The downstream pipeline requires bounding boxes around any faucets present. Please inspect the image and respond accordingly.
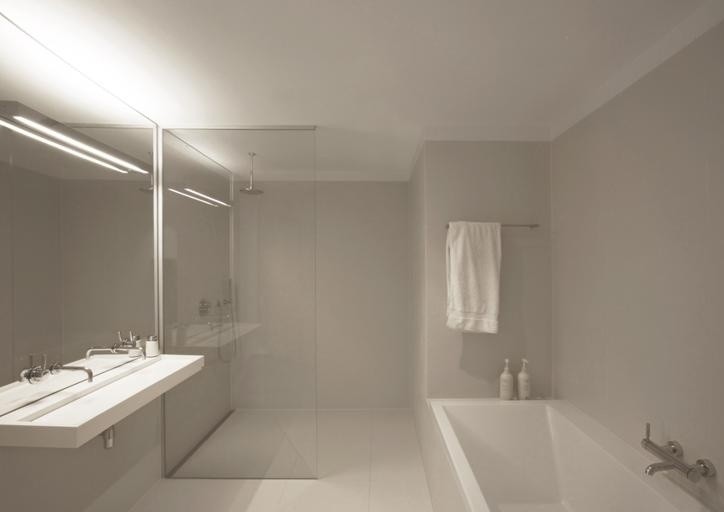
[637,421,716,484]
[208,322,223,331]
[115,343,146,359]
[20,362,94,383]
[85,345,118,359]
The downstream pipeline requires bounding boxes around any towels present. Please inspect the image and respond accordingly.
[443,217,502,334]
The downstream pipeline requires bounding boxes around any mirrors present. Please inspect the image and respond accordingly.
[161,127,232,354]
[0,9,154,394]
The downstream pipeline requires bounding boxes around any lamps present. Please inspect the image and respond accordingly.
[0,117,128,177]
[167,184,217,211]
[184,184,231,208]
[4,100,153,175]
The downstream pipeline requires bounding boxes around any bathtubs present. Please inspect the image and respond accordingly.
[427,398,723,512]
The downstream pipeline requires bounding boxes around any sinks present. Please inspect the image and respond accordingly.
[187,321,262,361]
[185,322,222,340]
[0,353,142,417]
[0,352,205,449]
